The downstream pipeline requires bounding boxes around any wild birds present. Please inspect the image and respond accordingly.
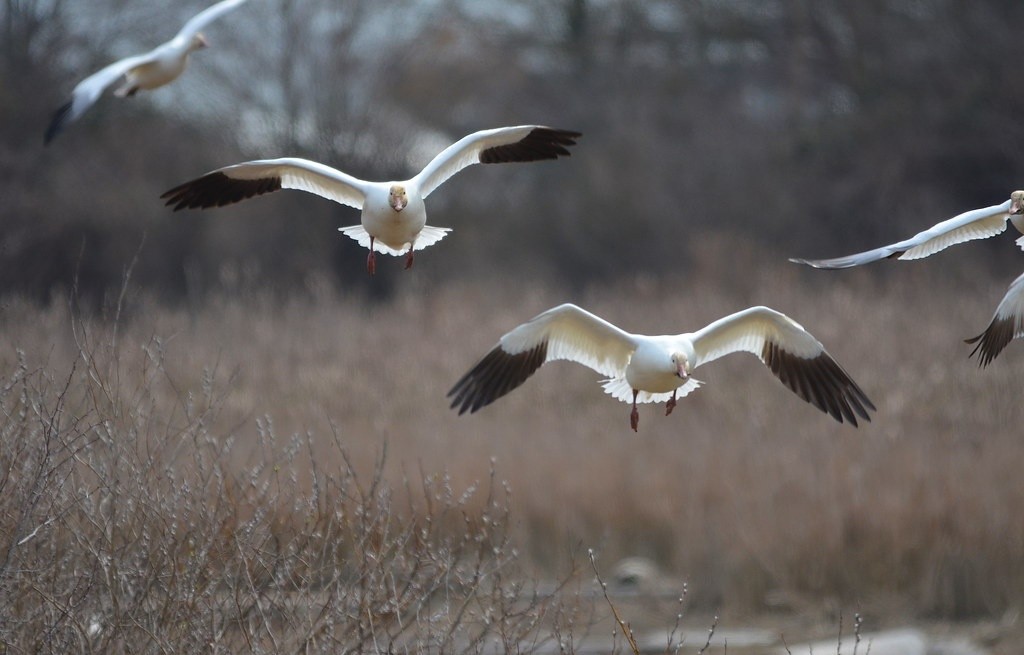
[41,0,249,148]
[160,125,583,278]
[446,303,877,430]
[789,190,1024,370]
